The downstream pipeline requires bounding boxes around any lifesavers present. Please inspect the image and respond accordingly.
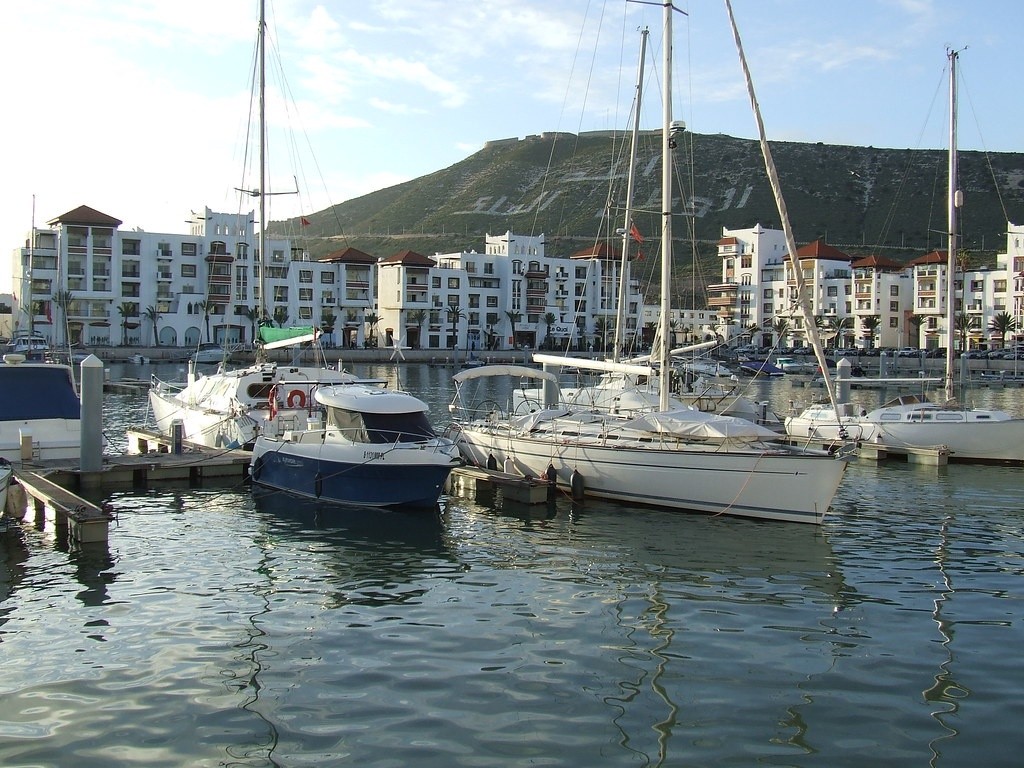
[287,390,306,408]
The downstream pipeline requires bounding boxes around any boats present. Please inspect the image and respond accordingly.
[5,329,50,354]
[126,352,151,364]
[245,378,464,514]
[188,342,233,365]
[0,456,14,513]
[0,195,109,461]
[68,352,89,364]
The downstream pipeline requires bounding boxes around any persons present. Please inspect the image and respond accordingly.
[171,336,191,345]
[90,335,110,346]
[128,336,139,344]
[472,340,475,351]
[219,337,239,343]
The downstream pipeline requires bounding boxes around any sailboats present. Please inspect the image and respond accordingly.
[426,1,861,526]
[146,0,409,453]
[783,44,1024,467]
[512,26,838,449]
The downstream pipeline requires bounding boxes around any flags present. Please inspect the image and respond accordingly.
[301,216,310,226]
[631,223,642,243]
[638,250,643,258]
